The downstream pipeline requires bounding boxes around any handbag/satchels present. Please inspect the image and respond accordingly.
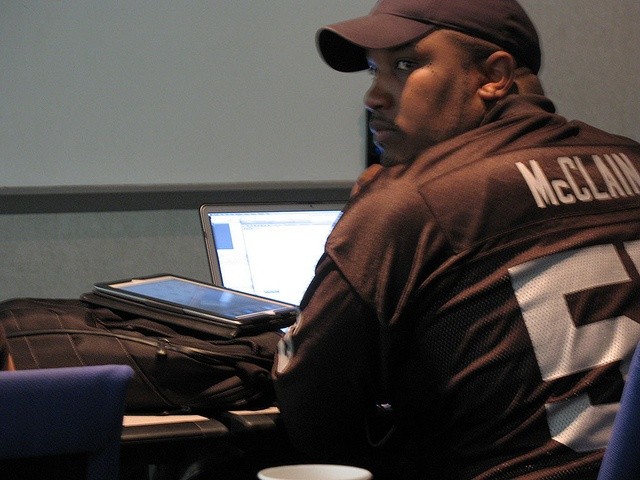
[1,298,286,417]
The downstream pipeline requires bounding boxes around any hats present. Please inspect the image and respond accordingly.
[314,0,541,75]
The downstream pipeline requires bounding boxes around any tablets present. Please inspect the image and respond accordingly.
[200,200,349,306]
[93,269,299,337]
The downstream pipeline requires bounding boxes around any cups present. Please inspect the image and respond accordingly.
[258,464,372,478]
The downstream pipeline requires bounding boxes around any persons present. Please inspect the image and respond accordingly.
[277,1,640,479]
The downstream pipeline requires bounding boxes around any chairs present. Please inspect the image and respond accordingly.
[598,343,639,478]
[0,365,134,480]
